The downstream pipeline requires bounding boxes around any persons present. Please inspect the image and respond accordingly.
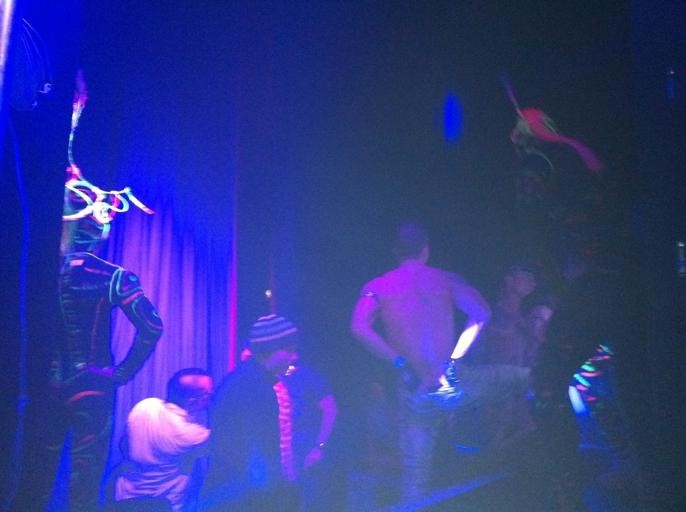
[3,107,686,510]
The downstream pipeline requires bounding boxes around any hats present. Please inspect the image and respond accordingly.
[247,313,299,354]
